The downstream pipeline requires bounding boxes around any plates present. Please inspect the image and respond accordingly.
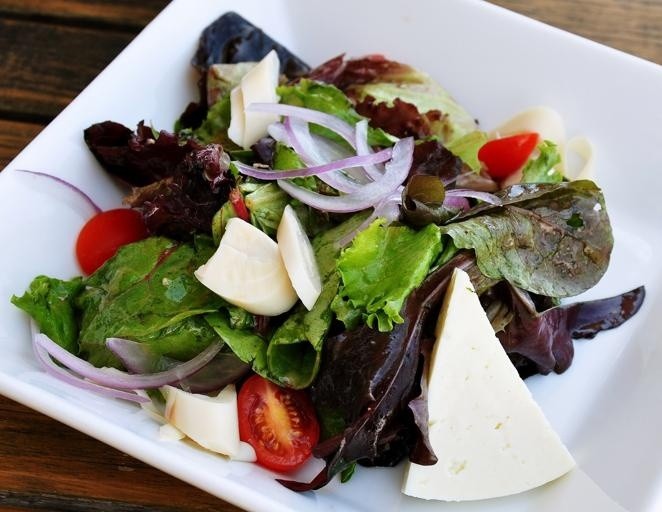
[2,1,662,509]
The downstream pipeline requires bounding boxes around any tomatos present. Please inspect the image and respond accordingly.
[76,208,149,276]
[477,133,540,180]
[236,375,320,471]
